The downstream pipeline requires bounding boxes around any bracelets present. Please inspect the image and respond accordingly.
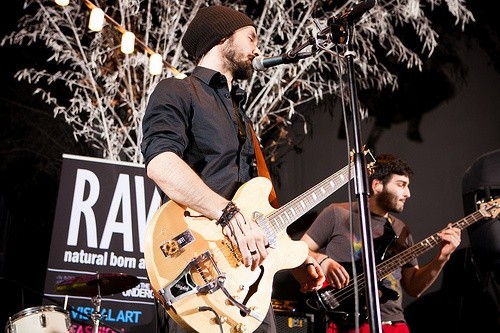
[217,200,246,253]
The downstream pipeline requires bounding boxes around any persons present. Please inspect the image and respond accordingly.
[139,6,326,333]
[299,157,461,333]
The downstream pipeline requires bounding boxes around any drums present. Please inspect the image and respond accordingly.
[5,306,72,333]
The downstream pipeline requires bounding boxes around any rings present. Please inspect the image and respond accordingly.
[250,250,258,255]
[265,242,269,248]
[305,263,314,269]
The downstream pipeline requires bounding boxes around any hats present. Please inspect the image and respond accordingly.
[182,6,257,63]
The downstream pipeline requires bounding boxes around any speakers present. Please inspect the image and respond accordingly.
[272,311,314,333]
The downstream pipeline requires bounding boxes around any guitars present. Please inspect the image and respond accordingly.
[314,195,500,328]
[144,145,379,333]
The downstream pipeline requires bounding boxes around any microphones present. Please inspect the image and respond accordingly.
[251,52,313,70]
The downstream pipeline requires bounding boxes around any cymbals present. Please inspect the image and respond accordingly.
[46,273,140,307]
